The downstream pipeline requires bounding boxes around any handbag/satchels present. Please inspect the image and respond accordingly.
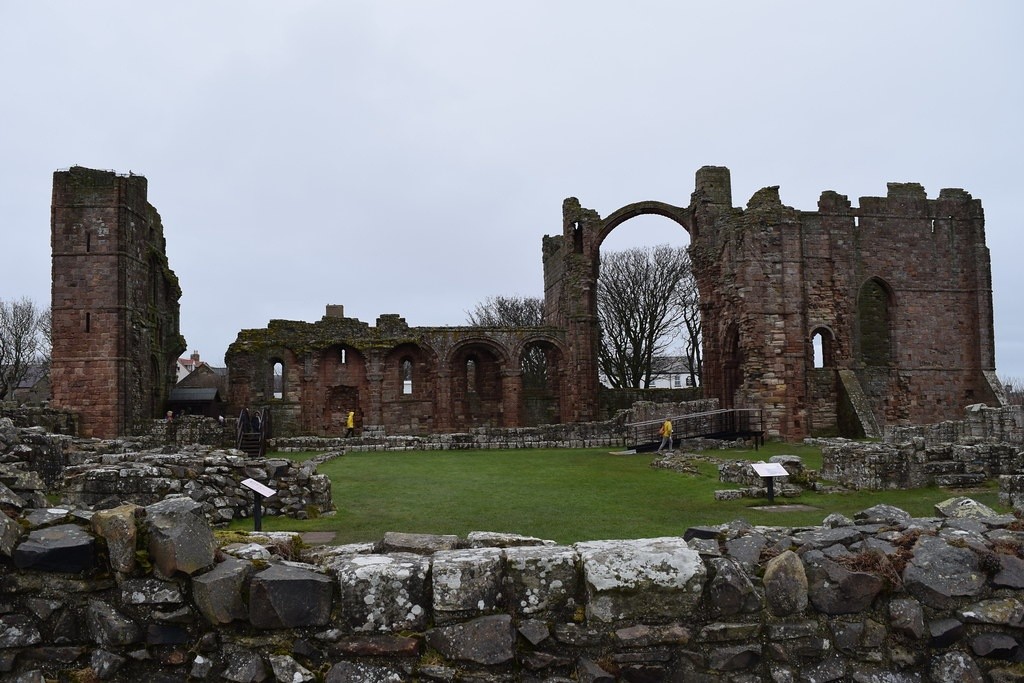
[659,426,665,434]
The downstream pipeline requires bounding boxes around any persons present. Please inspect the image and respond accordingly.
[251,410,261,432]
[166,409,185,420]
[656,418,675,455]
[242,406,252,433]
[344,411,354,438]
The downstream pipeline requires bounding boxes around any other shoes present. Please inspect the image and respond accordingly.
[657,452,662,456]
[670,450,674,453]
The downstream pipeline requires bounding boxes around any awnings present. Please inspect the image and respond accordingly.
[168,387,218,401]
